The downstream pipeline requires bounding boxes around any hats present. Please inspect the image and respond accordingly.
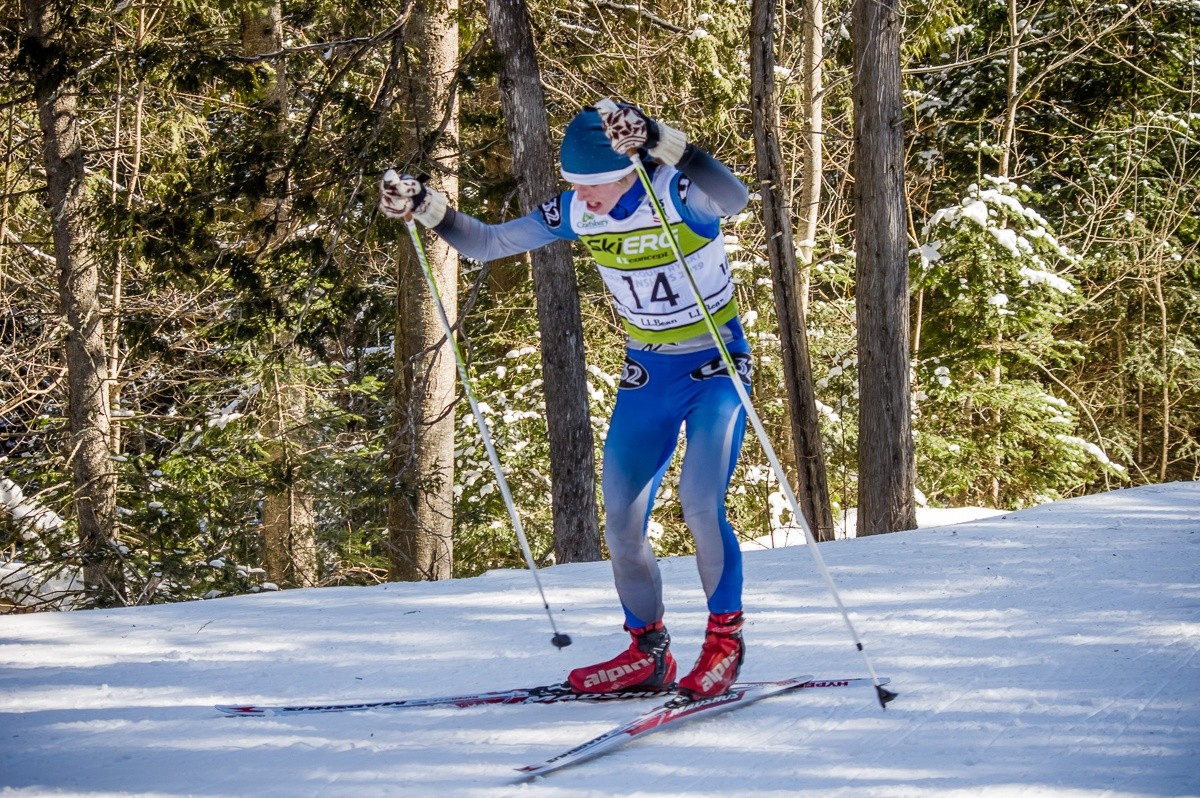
[560,105,636,184]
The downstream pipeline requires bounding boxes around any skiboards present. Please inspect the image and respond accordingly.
[213,675,890,775]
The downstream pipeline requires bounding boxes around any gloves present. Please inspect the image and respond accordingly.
[600,95,659,152]
[379,173,433,222]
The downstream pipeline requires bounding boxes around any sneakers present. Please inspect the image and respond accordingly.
[675,611,745,695]
[567,622,676,692]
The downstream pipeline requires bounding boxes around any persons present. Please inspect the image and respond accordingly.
[380,98,752,700]
